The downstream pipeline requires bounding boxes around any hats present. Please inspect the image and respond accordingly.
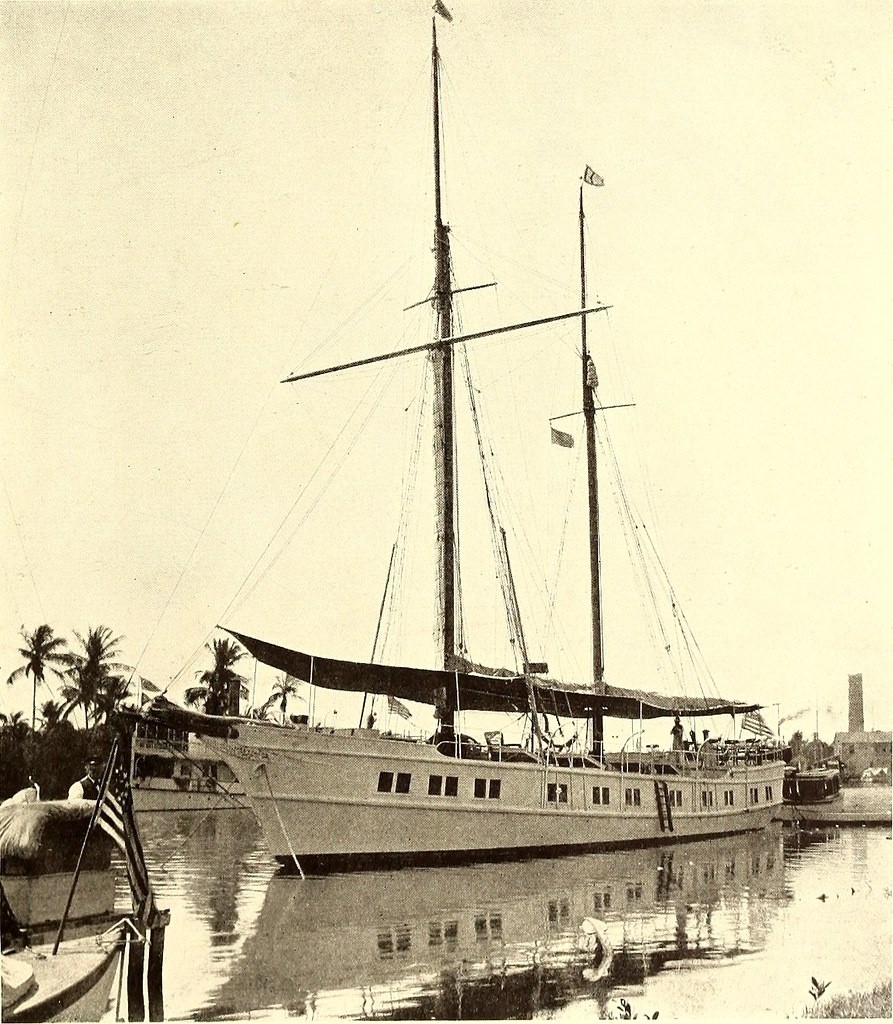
[85,757,103,765]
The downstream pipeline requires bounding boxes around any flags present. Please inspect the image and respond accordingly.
[387,695,412,720]
[140,693,151,705]
[550,428,574,449]
[139,675,161,692]
[741,708,775,740]
[93,739,163,931]
[582,164,605,187]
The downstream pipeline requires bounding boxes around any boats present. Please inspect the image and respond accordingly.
[0,916,140,1023]
[777,764,893,827]
[137,0,792,873]
[128,712,255,809]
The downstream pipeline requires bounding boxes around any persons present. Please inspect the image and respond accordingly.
[670,717,685,751]
[367,711,378,729]
[68,756,108,802]
